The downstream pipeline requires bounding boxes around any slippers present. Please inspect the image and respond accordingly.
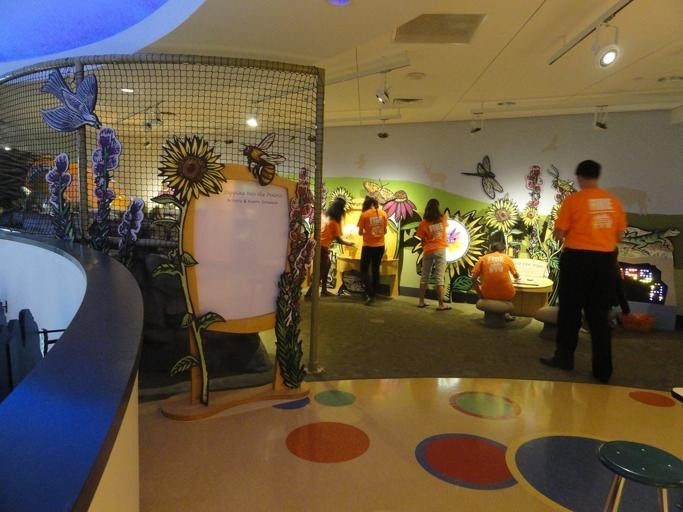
[436,305,452,311]
[417,302,431,308]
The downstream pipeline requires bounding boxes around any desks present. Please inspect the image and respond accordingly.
[512,277,553,317]
[335,255,399,297]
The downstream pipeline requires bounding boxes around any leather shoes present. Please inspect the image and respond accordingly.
[539,357,575,371]
[591,371,611,384]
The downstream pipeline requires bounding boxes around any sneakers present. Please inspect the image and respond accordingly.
[321,291,336,297]
[371,296,376,303]
[304,295,312,302]
[363,295,370,305]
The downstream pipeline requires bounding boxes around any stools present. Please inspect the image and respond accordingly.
[670,387,682,403]
[475,299,513,328]
[533,307,558,342]
[596,440,682,512]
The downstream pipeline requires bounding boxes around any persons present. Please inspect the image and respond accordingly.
[540,160,627,382]
[417,199,452,312]
[357,195,388,305]
[305,197,354,298]
[474,242,519,324]
[579,246,631,334]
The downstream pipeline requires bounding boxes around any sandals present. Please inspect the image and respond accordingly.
[504,314,516,322]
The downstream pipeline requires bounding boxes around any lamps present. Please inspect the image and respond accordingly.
[246,106,258,127]
[374,72,391,105]
[594,22,621,70]
